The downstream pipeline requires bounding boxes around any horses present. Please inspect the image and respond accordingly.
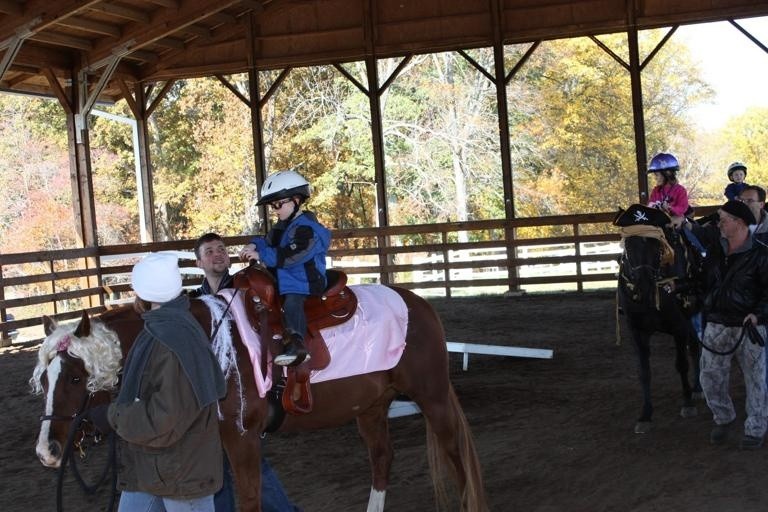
[28,285,489,512]
[617,221,720,434]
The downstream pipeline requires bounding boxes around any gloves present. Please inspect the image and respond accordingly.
[89,405,110,434]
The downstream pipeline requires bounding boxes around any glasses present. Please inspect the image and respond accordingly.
[271,199,291,209]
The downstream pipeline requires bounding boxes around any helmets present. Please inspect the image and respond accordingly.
[647,153,679,172]
[255,170,310,205]
[728,163,747,175]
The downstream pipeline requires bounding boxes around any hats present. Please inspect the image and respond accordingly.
[722,200,756,225]
[131,253,182,302]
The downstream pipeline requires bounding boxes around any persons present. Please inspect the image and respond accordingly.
[238,170,332,366]
[186,232,294,511]
[729,186,767,247]
[106,253,228,511]
[662,200,767,451]
[685,196,721,224]
[645,152,707,260]
[723,160,748,202]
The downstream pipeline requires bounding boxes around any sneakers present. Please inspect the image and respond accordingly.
[711,423,726,443]
[274,340,311,366]
[739,435,764,449]
